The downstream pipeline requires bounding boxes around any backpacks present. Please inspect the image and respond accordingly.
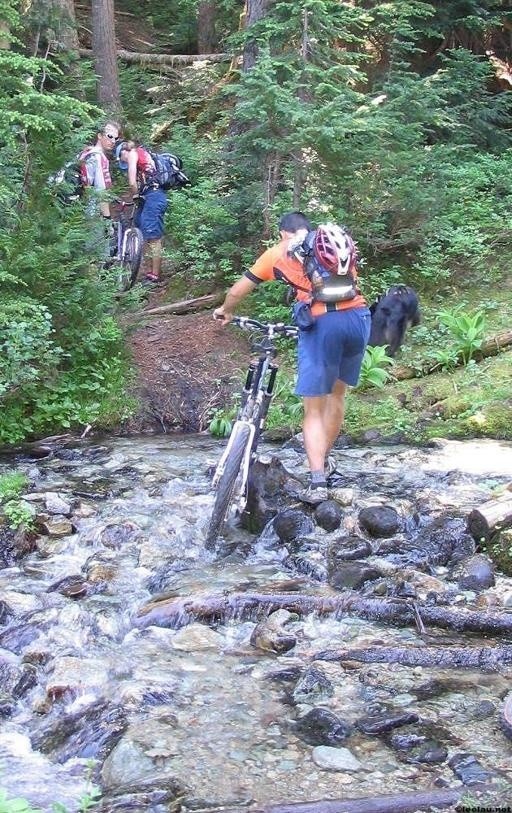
[137,145,183,190]
[52,150,98,202]
[287,230,358,304]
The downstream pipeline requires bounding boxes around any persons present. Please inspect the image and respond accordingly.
[212,211,373,504]
[114,138,168,284]
[77,121,121,280]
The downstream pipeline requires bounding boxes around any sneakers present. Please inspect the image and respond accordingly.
[297,482,329,505]
[146,271,161,282]
[304,454,338,485]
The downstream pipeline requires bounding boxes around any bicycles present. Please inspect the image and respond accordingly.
[104,194,146,292]
[205,313,299,548]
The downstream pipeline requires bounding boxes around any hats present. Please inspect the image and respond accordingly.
[115,142,130,170]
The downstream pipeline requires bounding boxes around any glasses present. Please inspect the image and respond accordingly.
[105,133,120,142]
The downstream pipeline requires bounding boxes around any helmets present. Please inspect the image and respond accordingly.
[161,153,182,170]
[312,223,357,277]
